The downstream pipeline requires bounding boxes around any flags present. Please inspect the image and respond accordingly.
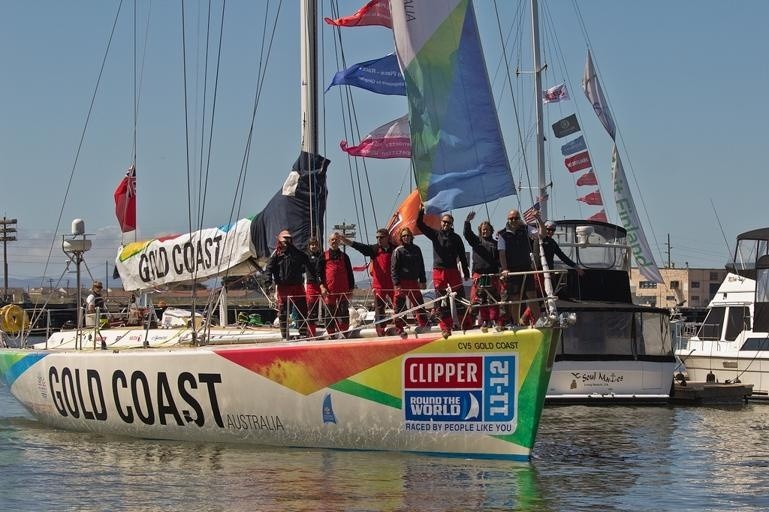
[522,203,540,224]
[114,166,136,233]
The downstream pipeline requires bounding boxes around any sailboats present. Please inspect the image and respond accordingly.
[0,0,563,466]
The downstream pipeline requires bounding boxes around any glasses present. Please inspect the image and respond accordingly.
[509,217,520,220]
[377,236,387,239]
[402,235,411,237]
[442,220,453,225]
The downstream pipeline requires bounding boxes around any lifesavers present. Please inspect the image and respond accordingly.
[0,305,30,334]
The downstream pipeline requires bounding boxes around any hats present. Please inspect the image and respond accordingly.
[93,281,103,288]
[279,230,291,238]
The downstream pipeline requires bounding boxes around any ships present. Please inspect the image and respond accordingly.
[663,226,769,397]
[519,0,677,404]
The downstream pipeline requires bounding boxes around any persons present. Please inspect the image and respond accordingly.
[238,300,296,330]
[392,227,430,336]
[75,282,169,329]
[265,231,314,341]
[336,228,400,337]
[315,233,355,339]
[467,208,583,333]
[418,205,472,338]
[301,237,325,337]
